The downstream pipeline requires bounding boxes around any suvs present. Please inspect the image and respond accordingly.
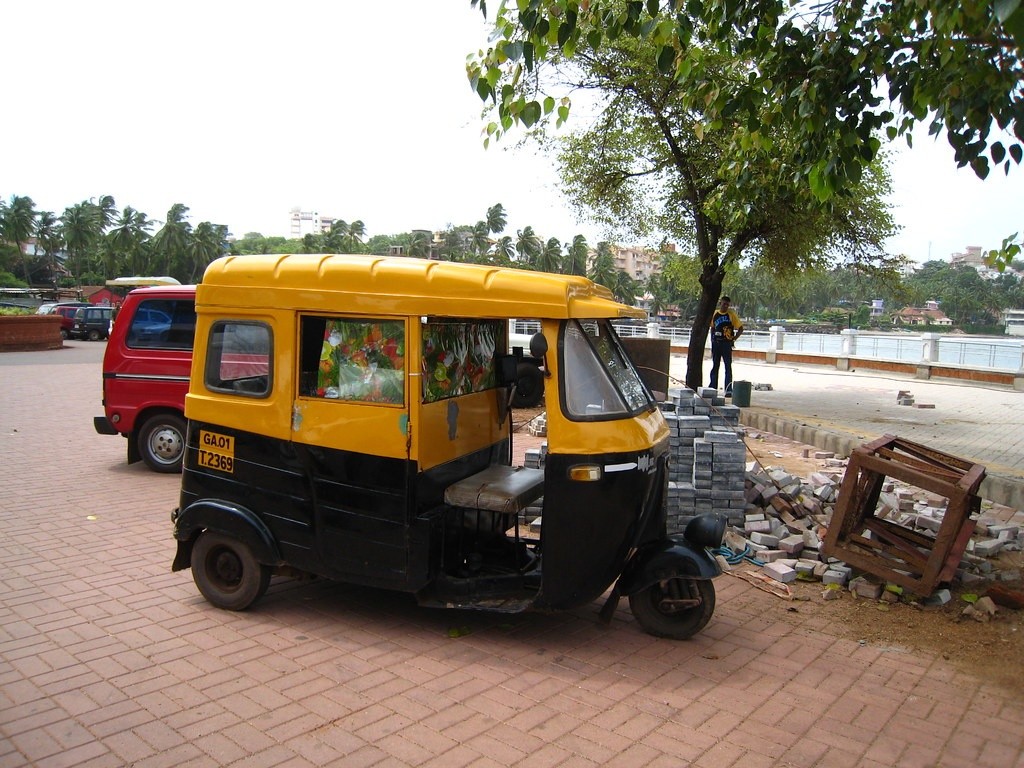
[33,302,116,341]
[94,284,268,474]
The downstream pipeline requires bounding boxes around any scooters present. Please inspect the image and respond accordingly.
[171,253,729,640]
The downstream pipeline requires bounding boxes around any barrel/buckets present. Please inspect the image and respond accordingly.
[732,381,751,406]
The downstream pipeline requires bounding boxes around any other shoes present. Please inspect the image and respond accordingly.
[725,391,732,397]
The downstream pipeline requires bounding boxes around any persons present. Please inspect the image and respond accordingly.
[709,296,743,397]
